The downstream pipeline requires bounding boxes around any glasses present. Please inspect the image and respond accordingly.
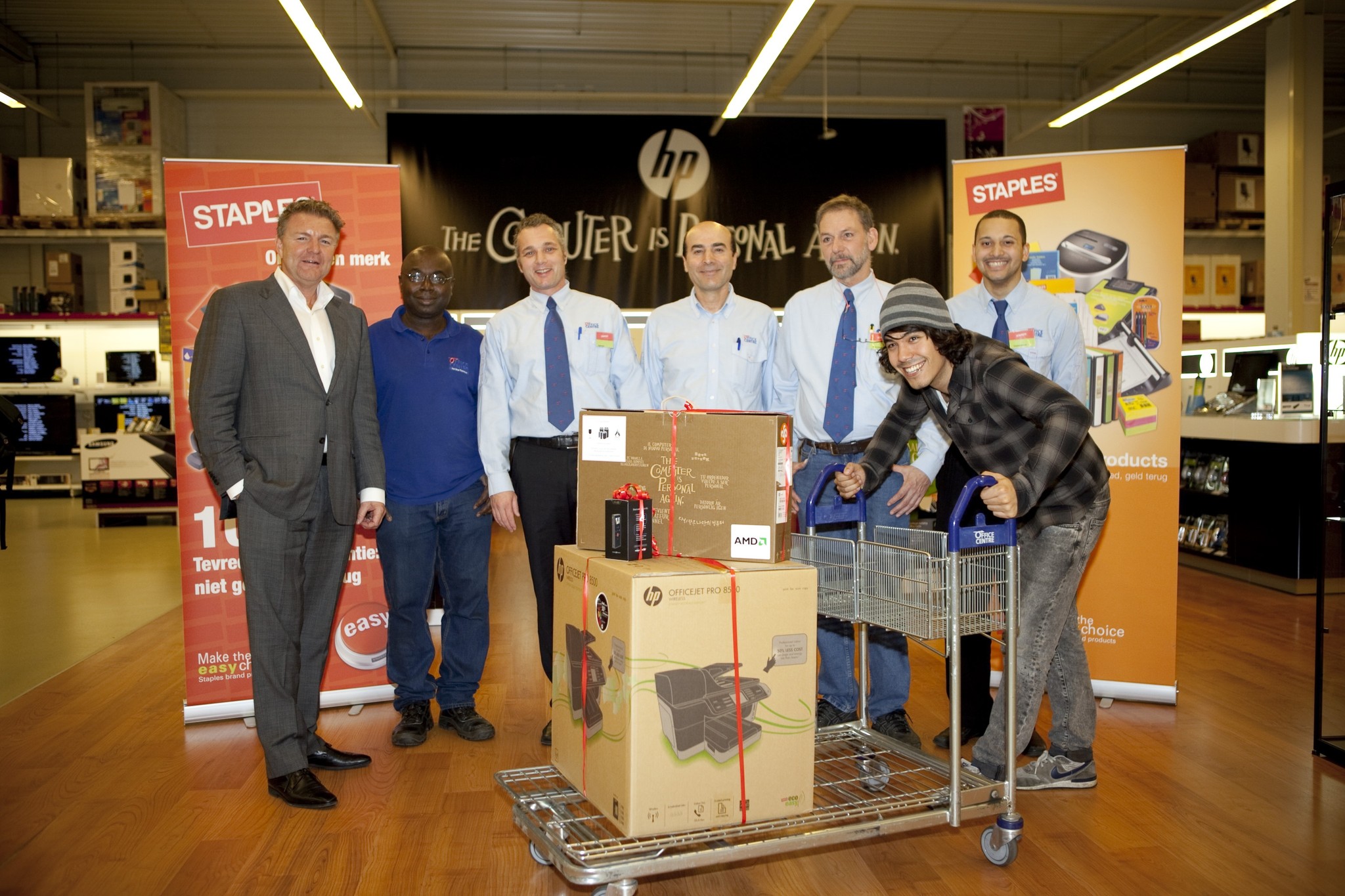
[401,271,452,286]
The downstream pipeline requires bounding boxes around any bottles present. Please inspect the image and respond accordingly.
[127,417,138,432]
[135,418,148,432]
[143,416,156,432]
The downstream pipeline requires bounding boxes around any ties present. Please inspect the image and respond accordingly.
[823,288,857,444]
[991,299,1010,349]
[544,296,575,432]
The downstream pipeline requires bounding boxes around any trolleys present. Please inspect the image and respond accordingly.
[489,460,1026,896]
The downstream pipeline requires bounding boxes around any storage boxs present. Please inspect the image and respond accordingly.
[109,242,146,268]
[49,284,82,311]
[111,292,146,312]
[1185,163,1216,225]
[45,250,83,284]
[110,266,146,290]
[135,279,163,300]
[1216,175,1265,220]
[550,545,817,838]
[603,497,652,561]
[1209,128,1266,177]
[1240,262,1265,307]
[576,408,793,562]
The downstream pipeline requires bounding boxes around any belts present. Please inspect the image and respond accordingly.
[802,437,873,456]
[321,452,328,466]
[514,433,579,450]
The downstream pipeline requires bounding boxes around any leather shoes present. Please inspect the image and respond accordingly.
[933,723,984,748]
[307,742,372,770]
[1021,729,1047,757]
[541,720,552,745]
[268,768,337,808]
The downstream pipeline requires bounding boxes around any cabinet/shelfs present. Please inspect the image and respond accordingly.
[0,228,167,325]
[0,313,160,493]
[1182,228,1344,321]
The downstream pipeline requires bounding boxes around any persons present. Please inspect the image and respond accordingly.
[932,209,1090,757]
[643,221,800,515]
[833,278,1112,808]
[476,211,651,751]
[771,195,953,755]
[189,201,386,810]
[368,245,496,746]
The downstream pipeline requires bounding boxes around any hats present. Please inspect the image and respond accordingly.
[879,278,958,342]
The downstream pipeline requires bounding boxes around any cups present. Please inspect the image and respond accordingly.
[1257,378,1276,415]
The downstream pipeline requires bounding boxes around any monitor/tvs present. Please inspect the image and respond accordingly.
[105,351,157,383]
[1227,352,1280,396]
[94,395,170,432]
[2,394,76,455]
[0,336,62,383]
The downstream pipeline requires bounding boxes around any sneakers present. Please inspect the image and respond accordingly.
[870,708,921,750]
[924,758,1002,810]
[438,706,495,740]
[391,699,433,746]
[816,698,859,728]
[1015,750,1097,790]
